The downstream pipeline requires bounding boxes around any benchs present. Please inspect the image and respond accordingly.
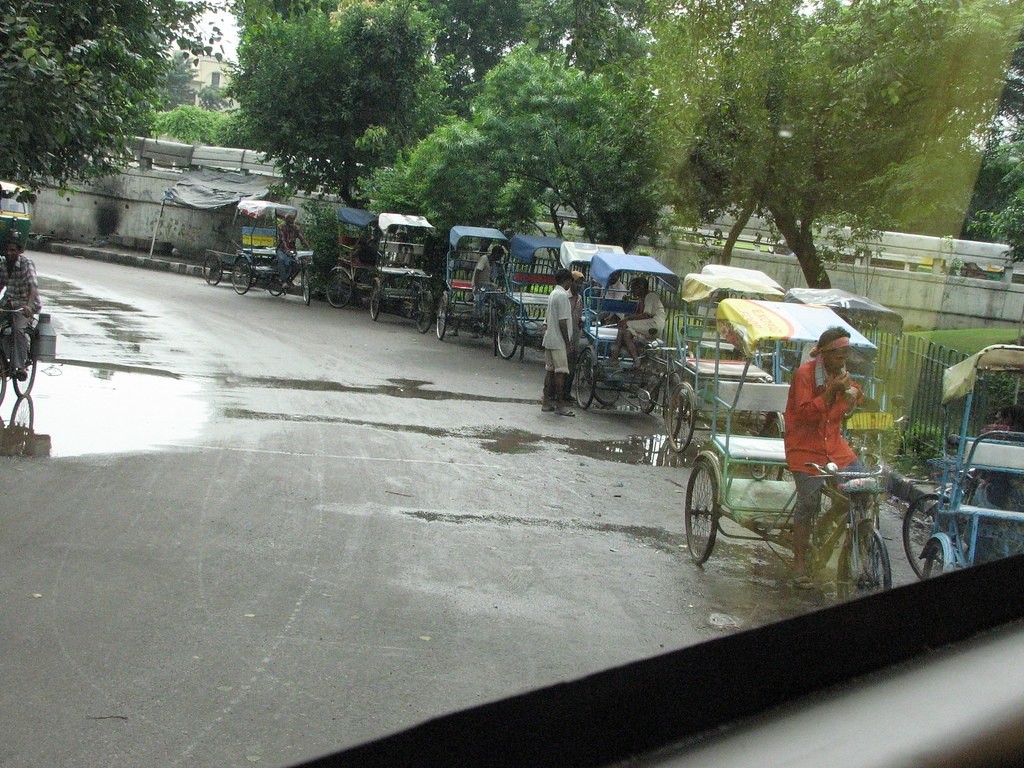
[686,300,736,352]
[241,227,277,255]
[378,241,426,275]
[448,259,477,289]
[506,271,557,305]
[711,380,791,466]
[937,437,1024,521]
[340,236,373,269]
[584,297,638,340]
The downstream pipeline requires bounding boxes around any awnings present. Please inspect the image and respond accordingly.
[942,343,1024,405]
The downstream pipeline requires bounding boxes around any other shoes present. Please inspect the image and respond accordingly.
[557,408,575,415]
[601,359,619,367]
[624,365,644,373]
[542,406,556,411]
[282,283,290,289]
[788,565,815,588]
[15,370,27,381]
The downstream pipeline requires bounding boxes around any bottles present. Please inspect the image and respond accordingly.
[838,477,877,490]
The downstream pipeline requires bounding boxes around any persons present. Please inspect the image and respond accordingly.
[781,328,865,590]
[276,212,310,287]
[542,268,666,417]
[963,405,1024,561]
[0,239,42,381]
[470,246,506,339]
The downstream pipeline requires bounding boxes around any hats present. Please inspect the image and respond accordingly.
[571,271,585,281]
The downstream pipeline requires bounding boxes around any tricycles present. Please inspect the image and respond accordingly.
[666,272,792,482]
[436,226,511,356]
[325,207,395,314]
[230,199,311,306]
[773,287,904,530]
[369,212,435,334]
[700,264,787,294]
[902,343,1024,580]
[685,299,893,603]
[560,241,627,404]
[495,234,580,369]
[201,248,280,290]
[576,253,684,439]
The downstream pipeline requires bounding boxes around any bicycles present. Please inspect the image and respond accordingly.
[0,307,37,407]
[0,395,34,457]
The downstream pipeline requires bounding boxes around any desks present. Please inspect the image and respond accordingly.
[678,324,772,382]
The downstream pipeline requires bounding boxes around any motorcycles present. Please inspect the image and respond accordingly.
[0,181,31,257]
